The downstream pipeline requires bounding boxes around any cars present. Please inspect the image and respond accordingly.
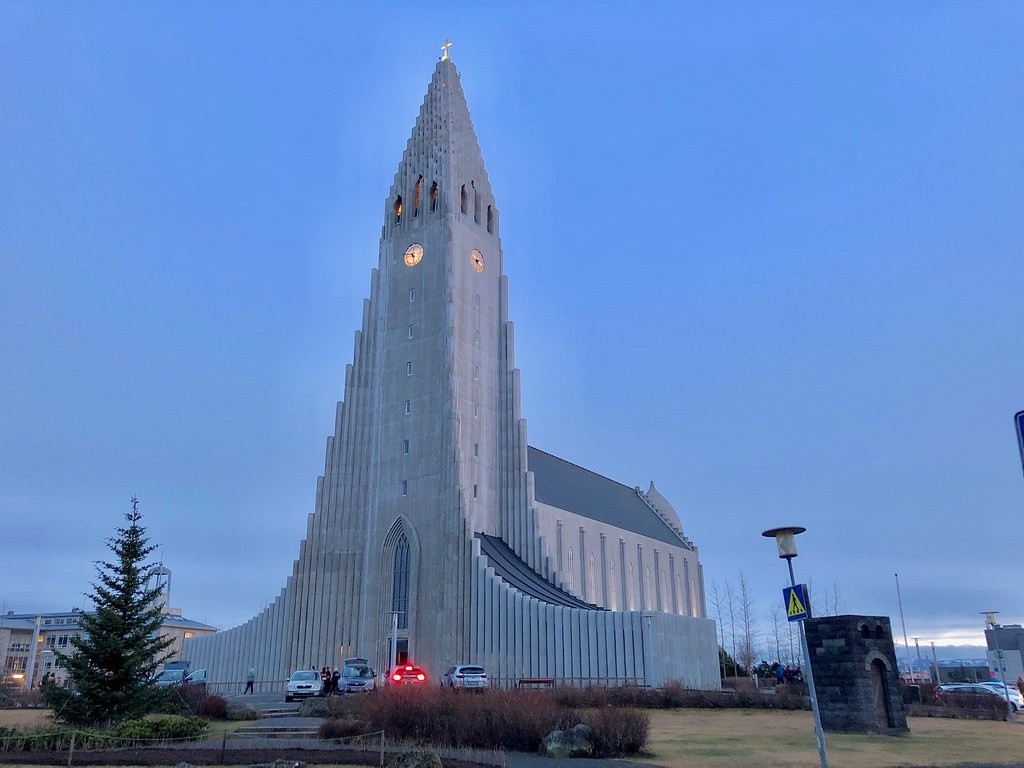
[285,670,326,702]
[148,659,207,692]
[931,679,1024,714]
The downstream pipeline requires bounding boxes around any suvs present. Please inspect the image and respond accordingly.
[439,665,487,694]
[391,665,427,689]
[337,658,377,695]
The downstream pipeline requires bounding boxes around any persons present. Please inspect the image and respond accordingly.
[1017,675,1024,698]
[243,668,255,695]
[41,672,56,692]
[1010,684,1016,690]
[309,666,341,697]
[775,664,804,685]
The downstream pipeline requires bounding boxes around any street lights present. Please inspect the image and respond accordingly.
[386,611,405,688]
[928,640,941,686]
[762,526,830,768]
[910,637,925,684]
[979,611,1013,718]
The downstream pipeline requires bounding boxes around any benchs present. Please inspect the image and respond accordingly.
[515,679,554,689]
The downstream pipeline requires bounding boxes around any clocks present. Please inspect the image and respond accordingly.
[470,249,485,273]
[404,244,423,267]
